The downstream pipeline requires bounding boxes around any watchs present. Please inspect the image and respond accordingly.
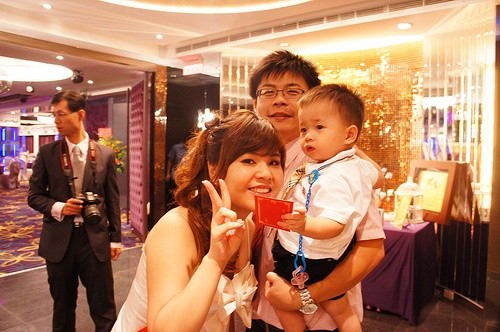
[298,288,318,315]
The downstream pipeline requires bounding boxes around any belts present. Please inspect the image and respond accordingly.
[73,221,84,229]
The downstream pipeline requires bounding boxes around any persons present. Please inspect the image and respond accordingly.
[27,90,124,332]
[243,51,386,332]
[166,133,192,206]
[110,108,286,332]
[270,83,385,332]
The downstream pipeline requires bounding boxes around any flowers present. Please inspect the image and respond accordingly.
[97,136,129,175]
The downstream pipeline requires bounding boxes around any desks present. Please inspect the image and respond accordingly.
[362,221,436,326]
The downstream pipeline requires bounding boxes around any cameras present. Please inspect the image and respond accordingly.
[76,191,102,224]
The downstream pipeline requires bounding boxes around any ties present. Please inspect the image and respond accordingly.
[266,164,306,239]
[72,146,84,198]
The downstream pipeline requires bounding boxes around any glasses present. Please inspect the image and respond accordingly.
[255,88,307,99]
[50,112,74,118]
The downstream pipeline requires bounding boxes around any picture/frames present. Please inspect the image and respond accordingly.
[408,159,459,225]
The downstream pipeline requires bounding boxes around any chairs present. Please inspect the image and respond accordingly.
[0,160,21,190]
[15,158,33,181]
[3,157,14,175]
